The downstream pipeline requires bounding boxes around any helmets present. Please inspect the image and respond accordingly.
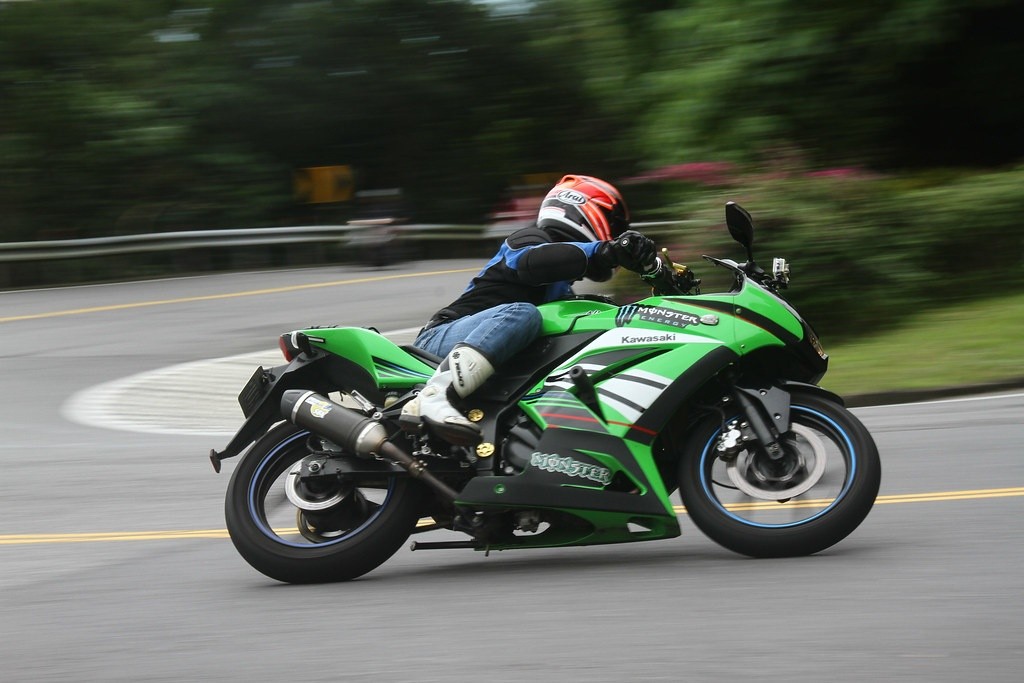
[537,174,630,282]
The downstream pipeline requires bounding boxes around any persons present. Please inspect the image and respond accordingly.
[399,174,658,444]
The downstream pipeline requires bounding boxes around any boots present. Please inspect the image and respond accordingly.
[398,342,495,447]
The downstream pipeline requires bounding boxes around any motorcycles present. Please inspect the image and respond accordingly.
[209,201,883,584]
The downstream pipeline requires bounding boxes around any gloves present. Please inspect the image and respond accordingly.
[597,230,656,274]
